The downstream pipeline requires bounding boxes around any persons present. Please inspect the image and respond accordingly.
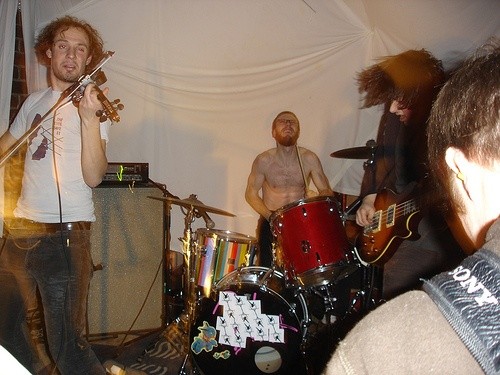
[355,49,447,280]
[244,111,333,268]
[321,43,499,374]
[0,15,108,375]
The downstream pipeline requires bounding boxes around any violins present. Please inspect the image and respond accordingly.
[71,69,124,123]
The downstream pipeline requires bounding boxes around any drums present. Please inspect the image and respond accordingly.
[193,228,256,298]
[189,267,308,375]
[269,196,356,288]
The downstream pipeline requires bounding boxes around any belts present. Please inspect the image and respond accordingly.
[30,221,91,234]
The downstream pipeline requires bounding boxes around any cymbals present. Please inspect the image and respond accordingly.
[330,146,375,159]
[147,195,236,218]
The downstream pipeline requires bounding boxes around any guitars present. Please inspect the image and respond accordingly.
[353,187,443,266]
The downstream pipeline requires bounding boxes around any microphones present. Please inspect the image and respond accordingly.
[189,195,215,229]
[93,262,106,271]
